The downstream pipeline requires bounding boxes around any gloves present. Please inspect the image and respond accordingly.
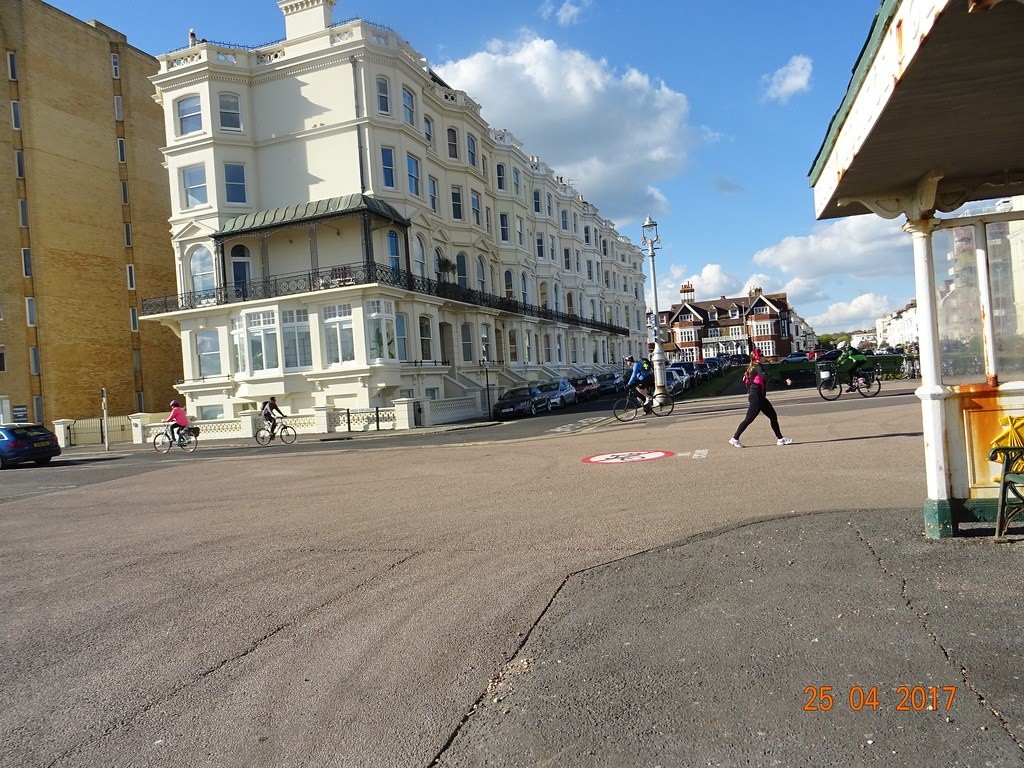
[837,364,840,368]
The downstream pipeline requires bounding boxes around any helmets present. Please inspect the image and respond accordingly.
[625,356,633,361]
[837,341,846,349]
[170,400,178,405]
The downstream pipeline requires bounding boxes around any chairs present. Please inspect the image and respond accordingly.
[989,446,1024,543]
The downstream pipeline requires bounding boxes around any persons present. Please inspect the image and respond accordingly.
[728,348,795,448]
[872,343,919,355]
[625,355,655,416]
[262,397,287,440]
[833,341,867,393]
[162,400,188,442]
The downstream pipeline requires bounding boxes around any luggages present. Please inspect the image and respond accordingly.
[643,396,652,405]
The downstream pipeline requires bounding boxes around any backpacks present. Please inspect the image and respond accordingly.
[261,401,271,411]
[635,358,650,373]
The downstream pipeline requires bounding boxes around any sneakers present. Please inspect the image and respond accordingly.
[777,438,793,446]
[729,438,744,448]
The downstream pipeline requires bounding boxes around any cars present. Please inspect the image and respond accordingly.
[0,422,62,470]
[779,348,884,363]
[492,371,645,421]
[649,353,750,397]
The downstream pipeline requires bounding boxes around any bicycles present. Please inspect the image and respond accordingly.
[894,349,983,380]
[613,382,675,422]
[153,420,198,454]
[818,362,881,401]
[254,416,297,445]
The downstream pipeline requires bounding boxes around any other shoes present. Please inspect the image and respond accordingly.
[846,387,856,392]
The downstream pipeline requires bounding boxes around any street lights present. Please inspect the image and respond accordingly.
[640,214,672,407]
